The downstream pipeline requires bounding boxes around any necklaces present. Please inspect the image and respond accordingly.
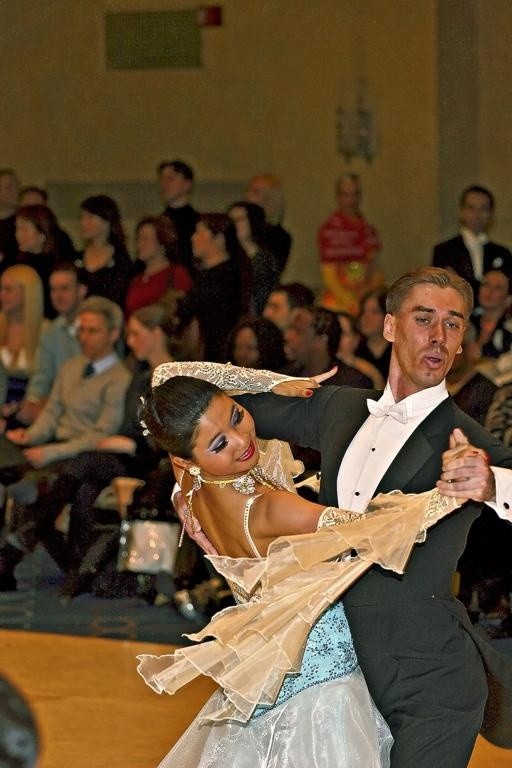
[197,470,256,495]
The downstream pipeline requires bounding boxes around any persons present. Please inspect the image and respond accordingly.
[229,267,511,767]
[137,360,489,768]
[1,161,511,639]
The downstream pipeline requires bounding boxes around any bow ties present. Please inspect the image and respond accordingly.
[367,399,406,423]
